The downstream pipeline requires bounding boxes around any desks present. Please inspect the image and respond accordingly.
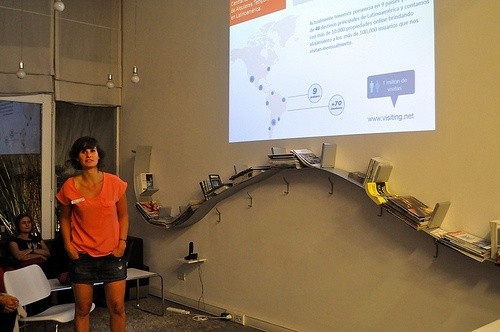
[47,268,165,317]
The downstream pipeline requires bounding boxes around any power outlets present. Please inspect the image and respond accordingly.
[178,272,185,280]
[228,311,245,325]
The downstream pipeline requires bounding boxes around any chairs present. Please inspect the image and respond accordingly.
[3,265,95,332]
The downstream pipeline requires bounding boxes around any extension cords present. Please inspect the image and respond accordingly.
[167,307,190,314]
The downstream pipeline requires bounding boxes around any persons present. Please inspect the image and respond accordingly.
[8,212,58,305]
[55,135,130,332]
[0,267,20,332]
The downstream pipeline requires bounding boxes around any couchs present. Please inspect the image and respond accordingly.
[1,236,143,304]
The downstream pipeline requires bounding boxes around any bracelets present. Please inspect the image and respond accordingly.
[119,238,127,242]
[31,248,34,253]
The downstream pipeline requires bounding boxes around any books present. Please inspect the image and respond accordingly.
[135,148,500,262]
